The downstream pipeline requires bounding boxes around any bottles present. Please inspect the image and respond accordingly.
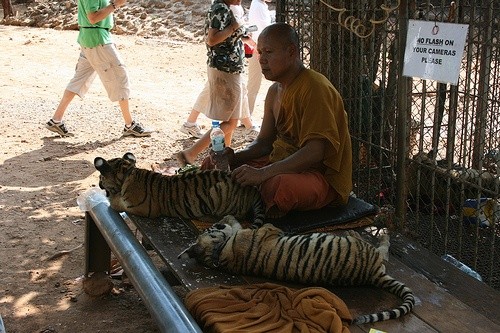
[210,121,231,172]
[440,253,483,283]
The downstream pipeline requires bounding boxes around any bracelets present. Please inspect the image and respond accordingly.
[109,1,117,9]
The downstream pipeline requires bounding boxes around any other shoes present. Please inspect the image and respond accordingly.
[177,151,191,169]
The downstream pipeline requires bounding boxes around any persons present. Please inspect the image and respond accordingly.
[197,23,353,217]
[44,0,150,137]
[172,0,271,171]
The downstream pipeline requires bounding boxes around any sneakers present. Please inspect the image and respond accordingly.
[245,125,261,142]
[45,118,68,136]
[180,124,203,139]
[123,120,150,137]
